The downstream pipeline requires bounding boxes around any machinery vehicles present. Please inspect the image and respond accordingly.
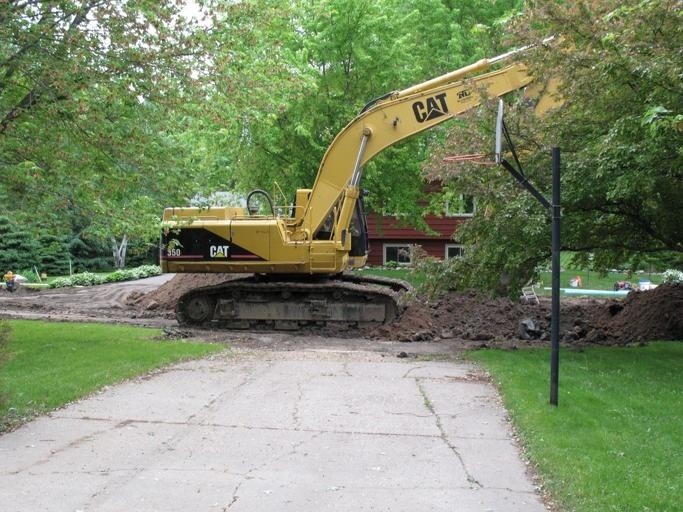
[156,26,584,337]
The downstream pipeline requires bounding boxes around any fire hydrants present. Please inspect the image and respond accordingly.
[2,271,17,292]
